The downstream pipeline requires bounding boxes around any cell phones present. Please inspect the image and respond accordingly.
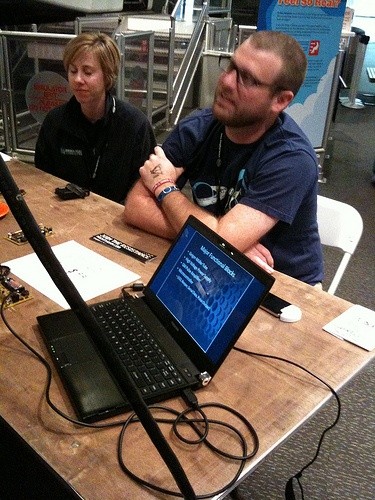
[259,292,291,317]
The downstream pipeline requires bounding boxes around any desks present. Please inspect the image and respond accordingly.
[0,156,375,500]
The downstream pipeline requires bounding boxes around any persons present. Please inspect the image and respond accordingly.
[122,29,322,286]
[36,34,156,207]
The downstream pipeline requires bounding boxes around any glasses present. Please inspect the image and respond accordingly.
[218,54,286,92]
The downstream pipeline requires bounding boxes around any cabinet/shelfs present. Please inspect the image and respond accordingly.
[0,30,155,163]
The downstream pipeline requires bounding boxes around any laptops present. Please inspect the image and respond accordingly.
[35,215,275,425]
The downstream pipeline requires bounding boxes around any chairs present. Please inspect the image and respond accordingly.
[298,195,364,293]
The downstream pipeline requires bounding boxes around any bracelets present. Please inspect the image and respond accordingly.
[158,186,179,202]
[153,178,173,193]
